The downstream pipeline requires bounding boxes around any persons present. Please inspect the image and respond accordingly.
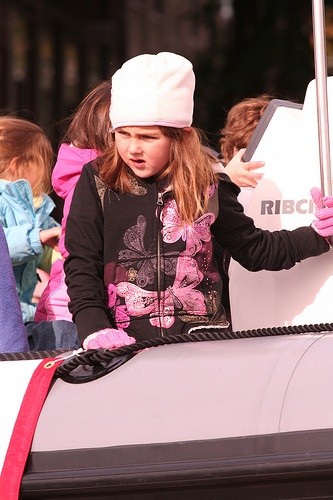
[63,53,332,354]
[0,81,281,357]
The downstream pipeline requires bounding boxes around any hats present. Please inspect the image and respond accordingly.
[108,52,196,128]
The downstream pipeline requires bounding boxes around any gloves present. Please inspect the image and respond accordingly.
[310,187,333,241]
[83,328,136,350]
